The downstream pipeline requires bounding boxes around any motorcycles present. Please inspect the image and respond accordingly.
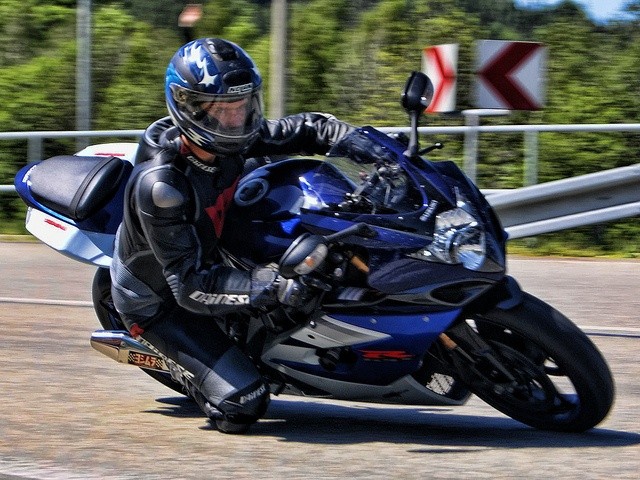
[14,71,614,433]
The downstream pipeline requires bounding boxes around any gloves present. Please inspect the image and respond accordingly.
[251,265,329,314]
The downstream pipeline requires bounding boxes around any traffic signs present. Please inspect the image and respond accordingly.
[421,44,459,113]
[471,39,547,111]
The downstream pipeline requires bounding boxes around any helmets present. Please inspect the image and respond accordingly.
[164,38,264,158]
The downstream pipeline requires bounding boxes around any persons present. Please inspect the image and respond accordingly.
[109,38,411,433]
